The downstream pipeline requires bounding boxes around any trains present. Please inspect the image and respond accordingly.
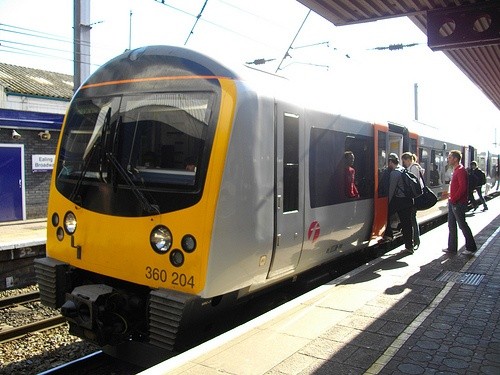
[32,44,500,368]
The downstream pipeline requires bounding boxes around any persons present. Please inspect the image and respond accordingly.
[401,152,425,249]
[442,150,477,255]
[470,161,488,211]
[385,152,414,253]
[328,151,359,202]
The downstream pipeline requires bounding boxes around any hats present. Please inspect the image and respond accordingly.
[388,153,399,160]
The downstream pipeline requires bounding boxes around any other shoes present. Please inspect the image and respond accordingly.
[400,249,413,255]
[383,231,393,241]
[472,206,478,212]
[481,207,488,212]
[462,247,477,255]
[442,247,457,254]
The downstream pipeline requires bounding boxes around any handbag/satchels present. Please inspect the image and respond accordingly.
[409,186,437,210]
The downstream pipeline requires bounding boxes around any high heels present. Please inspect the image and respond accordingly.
[396,222,403,232]
[412,238,420,249]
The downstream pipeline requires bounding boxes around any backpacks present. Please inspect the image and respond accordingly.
[472,167,486,185]
[391,168,422,198]
